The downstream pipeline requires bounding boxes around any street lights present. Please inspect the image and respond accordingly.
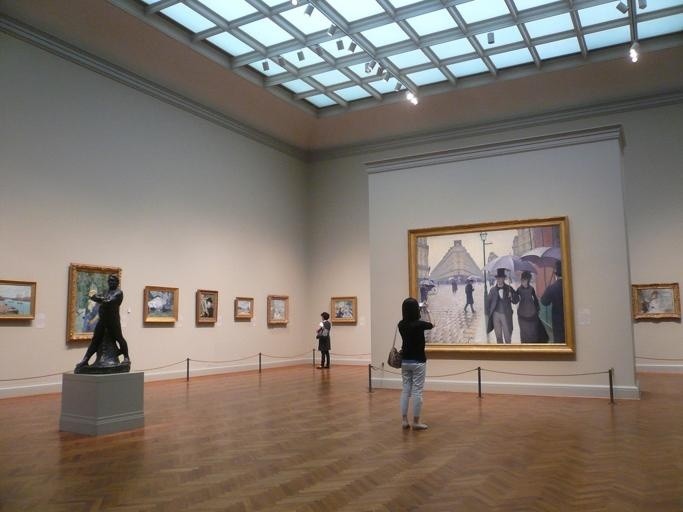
[478,231,488,314]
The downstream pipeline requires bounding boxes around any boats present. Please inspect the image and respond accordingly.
[0,296,22,315]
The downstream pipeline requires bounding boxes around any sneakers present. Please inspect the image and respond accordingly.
[400,420,410,429]
[411,421,428,430]
[316,365,330,369]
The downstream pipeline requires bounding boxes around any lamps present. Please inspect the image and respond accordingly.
[292,0,419,104]
[486,30,495,44]
[249,30,346,72]
[616,0,647,64]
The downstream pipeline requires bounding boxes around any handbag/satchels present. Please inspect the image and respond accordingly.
[315,328,329,339]
[386,346,403,369]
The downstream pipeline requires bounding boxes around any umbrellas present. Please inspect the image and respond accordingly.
[520,246,561,286]
[467,275,480,288]
[480,255,538,298]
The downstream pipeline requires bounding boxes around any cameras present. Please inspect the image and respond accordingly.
[419,301,429,311]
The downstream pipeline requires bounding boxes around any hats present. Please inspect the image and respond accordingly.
[553,260,561,277]
[494,268,507,278]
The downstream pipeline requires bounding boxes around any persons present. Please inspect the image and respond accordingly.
[397,298,435,430]
[540,260,565,343]
[82,289,100,332]
[485,268,519,344]
[75,274,131,368]
[418,280,477,314]
[201,297,214,317]
[315,312,332,369]
[516,271,549,343]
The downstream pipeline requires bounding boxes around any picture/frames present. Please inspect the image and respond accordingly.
[65,261,122,343]
[143,285,178,324]
[267,295,287,324]
[0,278,37,320]
[629,282,680,321]
[195,289,218,324]
[406,216,576,358]
[234,296,253,319]
[330,296,356,323]
